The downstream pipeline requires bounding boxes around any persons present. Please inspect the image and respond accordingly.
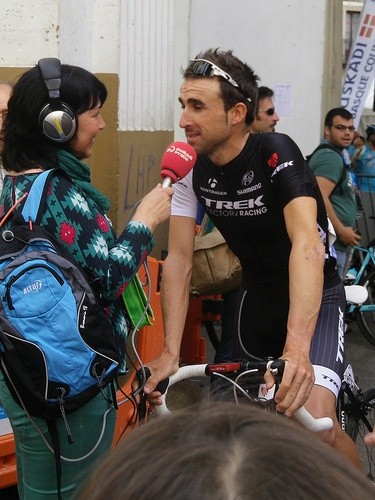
[74,402,375,500]
[0,57,176,500]
[131,44,374,471]
[0,82,12,195]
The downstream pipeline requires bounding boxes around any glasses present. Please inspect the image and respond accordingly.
[328,124,355,133]
[258,107,274,116]
[183,59,254,106]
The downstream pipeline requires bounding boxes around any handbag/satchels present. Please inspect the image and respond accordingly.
[122,274,155,329]
[0,167,124,421]
[190,229,242,299]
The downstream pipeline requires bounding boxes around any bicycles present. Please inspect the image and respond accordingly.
[136,358,375,483]
[340,228,375,348]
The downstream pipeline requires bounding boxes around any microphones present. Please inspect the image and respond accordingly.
[161,141,197,189]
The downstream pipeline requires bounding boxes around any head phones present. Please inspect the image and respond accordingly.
[38,58,78,144]
